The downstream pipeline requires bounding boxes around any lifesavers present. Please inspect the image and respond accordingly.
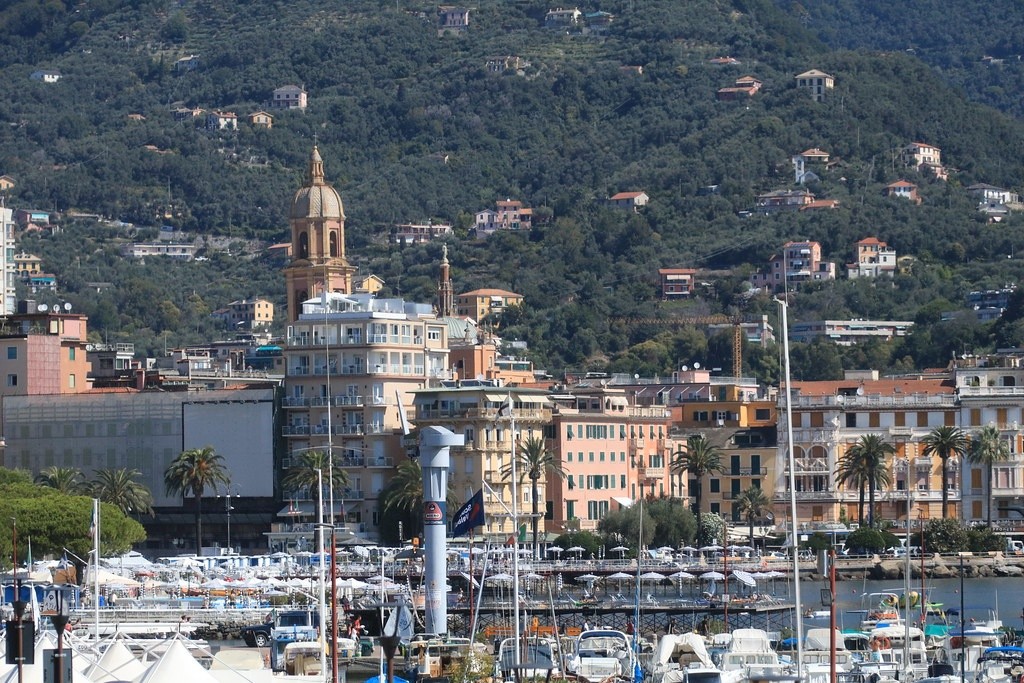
[877,637,890,649]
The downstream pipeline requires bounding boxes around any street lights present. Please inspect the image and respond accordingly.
[215,482,241,556]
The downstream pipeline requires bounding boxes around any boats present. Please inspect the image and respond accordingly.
[0,301,1024,682]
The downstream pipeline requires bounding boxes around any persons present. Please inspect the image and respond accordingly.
[340,594,368,646]
[100,590,118,609]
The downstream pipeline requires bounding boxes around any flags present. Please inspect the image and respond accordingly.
[452,488,485,539]
